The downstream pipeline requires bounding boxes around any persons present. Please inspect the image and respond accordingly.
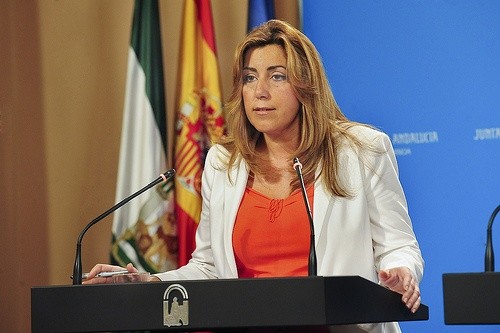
[81,17,425,333]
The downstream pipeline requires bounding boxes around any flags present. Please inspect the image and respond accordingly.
[246,0,274,34]
[176,0,232,271]
[108,0,177,277]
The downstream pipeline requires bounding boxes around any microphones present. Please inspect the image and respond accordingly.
[73,168,175,286]
[292,157,317,275]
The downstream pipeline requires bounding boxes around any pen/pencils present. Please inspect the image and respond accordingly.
[70,266,131,279]
